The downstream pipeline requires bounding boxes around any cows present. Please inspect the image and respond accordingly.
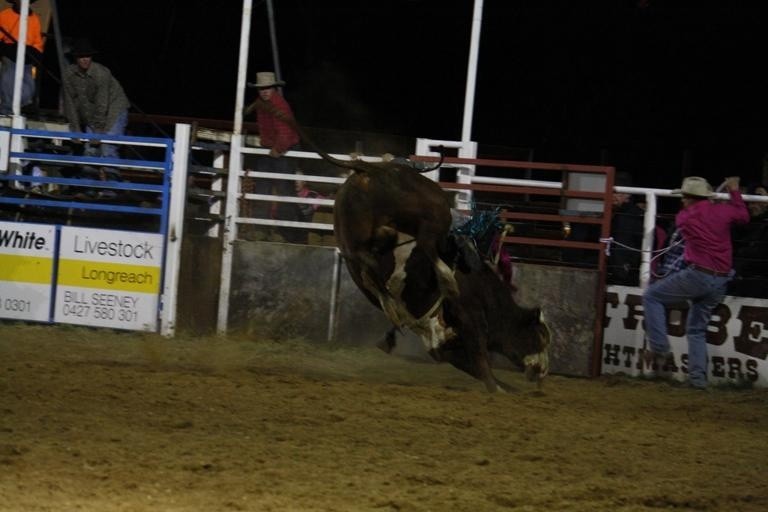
[245,97,553,396]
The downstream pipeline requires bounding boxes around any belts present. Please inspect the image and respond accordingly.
[695,266,729,277]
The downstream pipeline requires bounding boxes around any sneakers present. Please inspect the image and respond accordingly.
[245,230,287,243]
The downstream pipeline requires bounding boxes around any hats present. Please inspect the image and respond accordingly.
[67,42,96,56]
[248,73,284,88]
[672,176,717,199]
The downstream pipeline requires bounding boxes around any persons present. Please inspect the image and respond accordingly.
[641,176,751,390]
[271,166,326,236]
[243,71,309,244]
[544,175,767,299]
[60,38,131,198]
[0,0,68,194]
